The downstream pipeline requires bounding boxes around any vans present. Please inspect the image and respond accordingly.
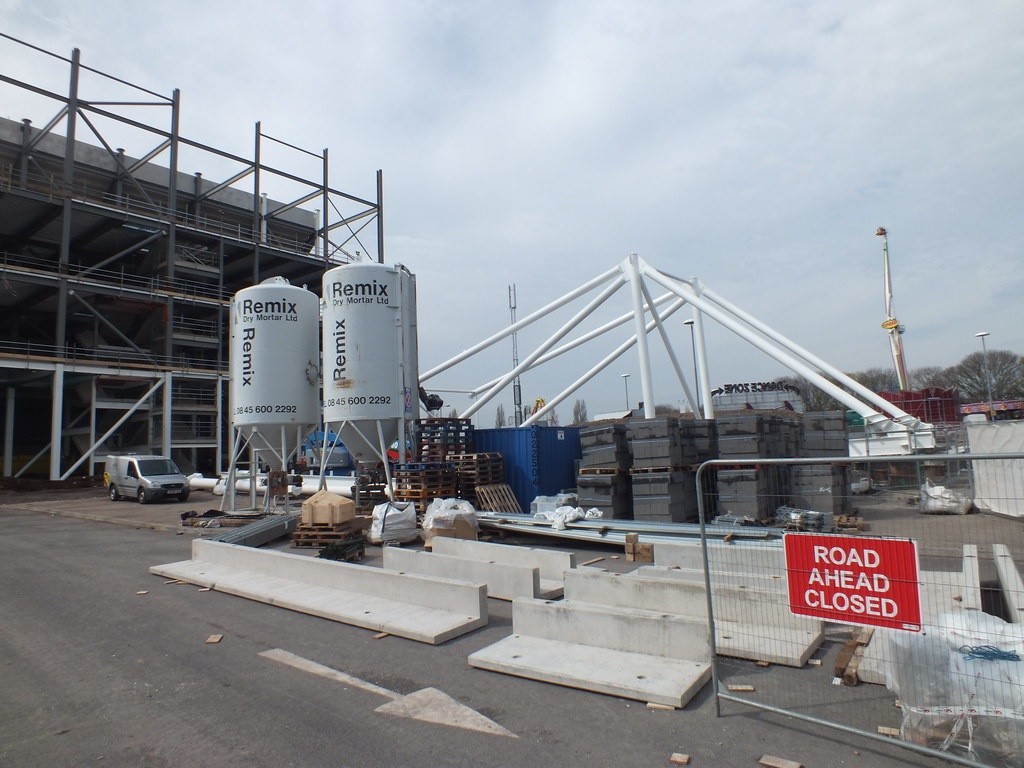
[103,453,191,504]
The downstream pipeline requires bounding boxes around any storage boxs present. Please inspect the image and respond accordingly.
[425,515,478,546]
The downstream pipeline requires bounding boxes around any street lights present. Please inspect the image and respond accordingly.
[620,373,631,411]
[683,318,701,409]
[975,332,995,421]
